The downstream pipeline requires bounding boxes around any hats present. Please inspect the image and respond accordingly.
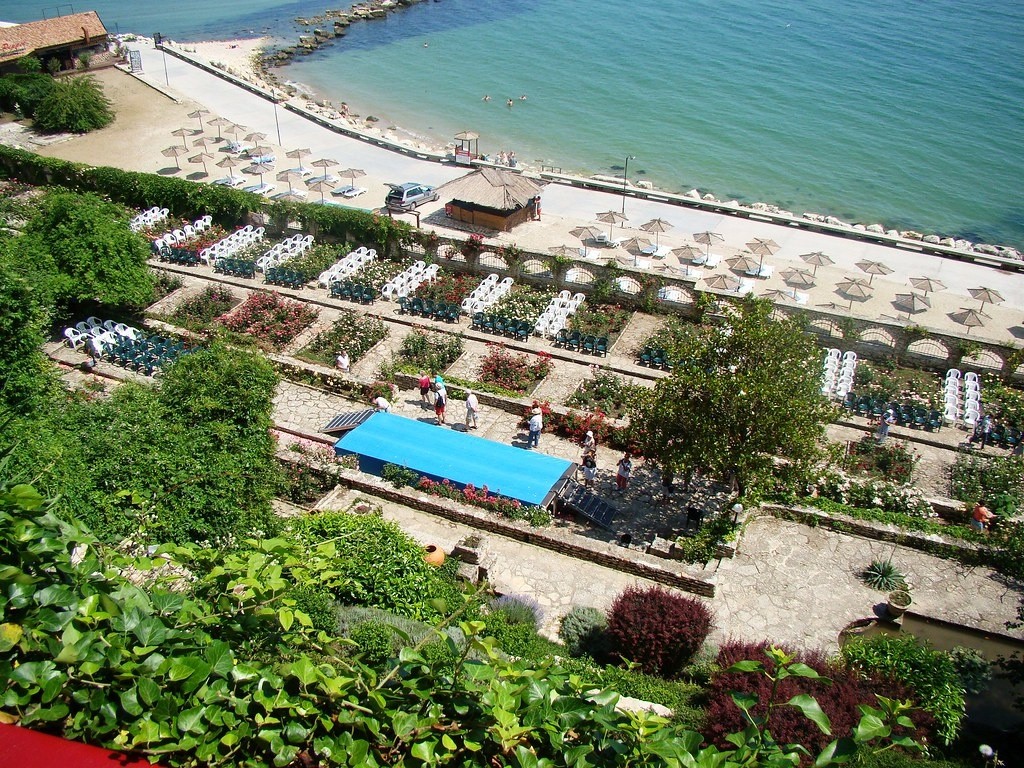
[462,389,471,394]
[435,382,442,389]
[886,408,894,416]
[586,431,594,437]
[624,454,630,458]
[431,370,438,375]
[531,408,541,415]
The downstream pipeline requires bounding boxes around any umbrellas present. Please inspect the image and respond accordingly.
[568,211,1006,335]
[162,110,366,203]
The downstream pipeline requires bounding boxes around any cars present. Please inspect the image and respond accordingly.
[383,182,440,212]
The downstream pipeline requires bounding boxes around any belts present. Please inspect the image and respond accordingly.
[385,404,390,410]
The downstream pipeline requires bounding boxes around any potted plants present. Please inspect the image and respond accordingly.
[886,584,912,618]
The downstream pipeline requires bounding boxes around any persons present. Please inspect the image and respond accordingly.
[579,431,597,491]
[418,370,447,426]
[337,350,350,373]
[970,500,996,535]
[661,466,674,499]
[877,409,894,435]
[80,336,97,366]
[968,413,990,451]
[615,454,632,494]
[525,400,543,449]
[369,396,391,413]
[460,389,479,433]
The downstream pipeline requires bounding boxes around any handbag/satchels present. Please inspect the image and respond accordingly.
[668,485,674,493]
[474,412,479,420]
[435,391,443,407]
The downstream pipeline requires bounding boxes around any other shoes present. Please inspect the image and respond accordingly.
[428,401,434,406]
[526,446,532,450]
[434,422,441,426]
[681,489,688,493]
[534,445,538,448]
[615,485,620,490]
[619,488,625,494]
[590,487,594,491]
[692,488,698,494]
[440,420,445,423]
[422,403,427,407]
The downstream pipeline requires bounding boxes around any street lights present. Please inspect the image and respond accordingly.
[622,155,635,228]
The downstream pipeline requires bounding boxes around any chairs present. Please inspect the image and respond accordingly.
[725,264,810,311]
[65,206,632,377]
[819,348,1024,454]
[216,139,367,205]
[636,234,674,269]
[675,252,723,279]
[636,345,714,377]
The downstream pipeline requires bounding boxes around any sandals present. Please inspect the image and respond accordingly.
[469,426,477,430]
[460,428,469,432]
